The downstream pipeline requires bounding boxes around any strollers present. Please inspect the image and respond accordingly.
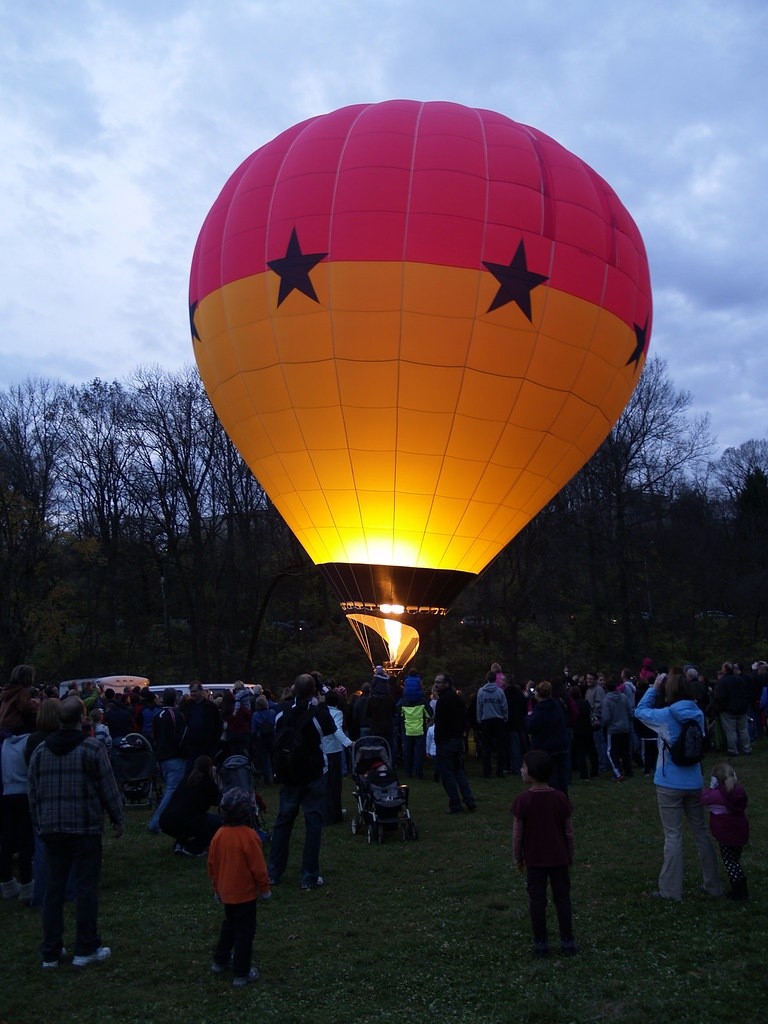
[109,732,163,811]
[211,748,269,828]
[347,736,420,844]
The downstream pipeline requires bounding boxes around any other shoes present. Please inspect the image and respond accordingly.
[560,939,574,955]
[536,943,549,958]
[700,885,723,896]
[723,750,739,758]
[467,805,478,814]
[611,775,624,783]
[741,750,752,756]
[171,843,181,854]
[653,890,683,903]
[445,806,465,814]
[184,848,208,858]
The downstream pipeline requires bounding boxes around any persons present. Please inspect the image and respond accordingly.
[634,671,722,904]
[269,666,476,890]
[510,751,574,959]
[472,658,768,779]
[0,664,276,901]
[700,765,751,901]
[28,696,125,969]
[208,796,272,987]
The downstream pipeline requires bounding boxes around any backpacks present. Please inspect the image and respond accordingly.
[271,700,324,784]
[662,716,706,777]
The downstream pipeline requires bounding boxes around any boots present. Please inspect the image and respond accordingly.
[728,877,749,900]
[18,879,36,900]
[2,877,21,899]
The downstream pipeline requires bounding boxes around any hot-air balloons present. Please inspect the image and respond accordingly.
[183,95,655,691]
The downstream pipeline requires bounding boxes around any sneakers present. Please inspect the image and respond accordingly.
[234,966,259,985]
[301,876,324,893]
[72,948,112,966]
[43,948,67,967]
[211,954,233,973]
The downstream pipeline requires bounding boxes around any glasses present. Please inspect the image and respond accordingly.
[434,680,446,684]
